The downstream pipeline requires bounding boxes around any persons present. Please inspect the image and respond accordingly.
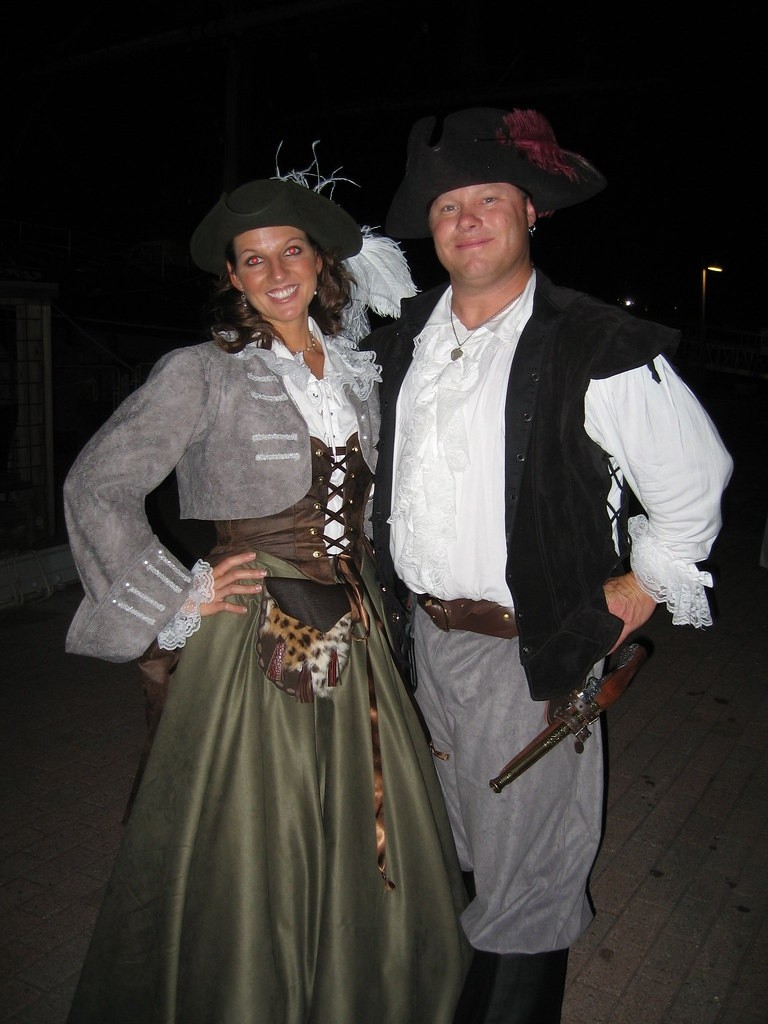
[351,106,734,1024]
[63,181,476,1024]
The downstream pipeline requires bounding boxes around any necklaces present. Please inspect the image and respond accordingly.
[451,289,524,362]
[291,331,316,354]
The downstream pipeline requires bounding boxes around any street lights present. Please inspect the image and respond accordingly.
[699,264,723,322]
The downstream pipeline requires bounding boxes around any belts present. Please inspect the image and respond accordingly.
[416,593,519,638]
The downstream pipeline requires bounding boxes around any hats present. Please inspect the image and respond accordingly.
[191,178,365,279]
[383,108,604,239]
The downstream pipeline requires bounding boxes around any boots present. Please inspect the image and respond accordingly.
[452,949,569,1024]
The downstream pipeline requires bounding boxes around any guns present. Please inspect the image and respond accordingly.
[489,642,648,794]
